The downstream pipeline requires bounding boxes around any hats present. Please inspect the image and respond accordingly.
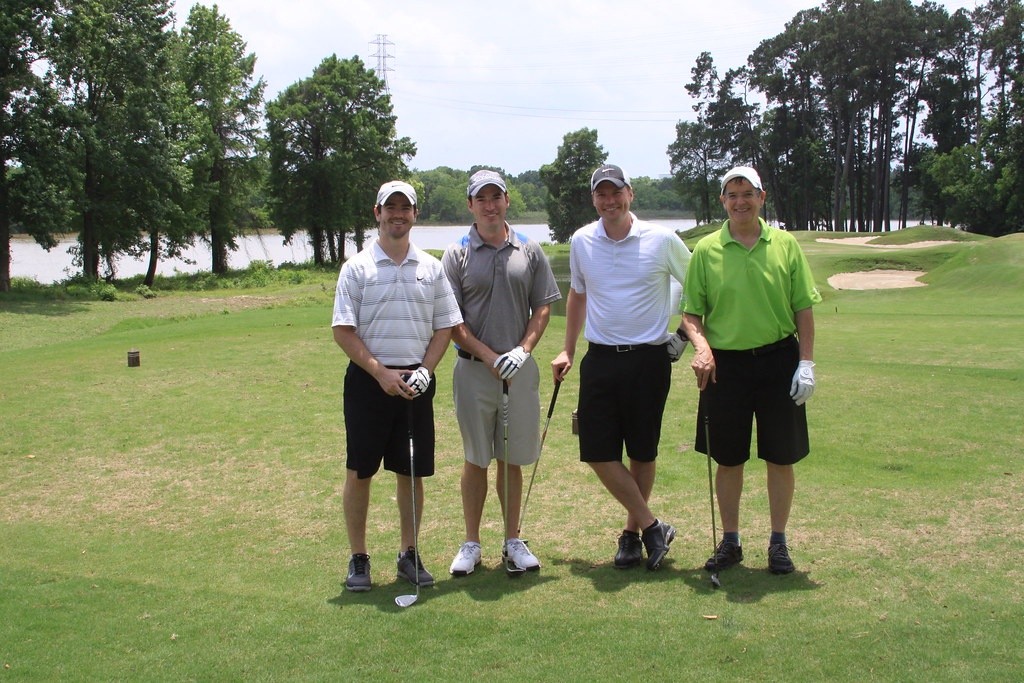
[376,181,418,211]
[721,165,763,196]
[466,170,507,199]
[590,164,633,194]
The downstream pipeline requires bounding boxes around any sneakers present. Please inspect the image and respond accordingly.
[704,538,744,572]
[768,540,795,574]
[642,518,677,570]
[397,546,436,587]
[502,538,542,571]
[346,553,372,591]
[450,542,482,576]
[614,534,643,569]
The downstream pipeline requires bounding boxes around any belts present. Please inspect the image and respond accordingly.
[457,350,484,363]
[588,341,665,353]
[711,335,796,357]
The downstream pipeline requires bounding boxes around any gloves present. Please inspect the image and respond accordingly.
[400,366,432,399]
[789,359,817,406]
[667,331,689,362]
[493,345,531,380]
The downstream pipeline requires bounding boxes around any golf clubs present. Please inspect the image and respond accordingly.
[503,378,525,577]
[394,374,420,607]
[703,389,720,588]
[517,368,564,544]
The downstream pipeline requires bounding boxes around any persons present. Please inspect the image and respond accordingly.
[679,166,823,575]
[551,164,693,571]
[331,180,464,593]
[439,169,562,574]
